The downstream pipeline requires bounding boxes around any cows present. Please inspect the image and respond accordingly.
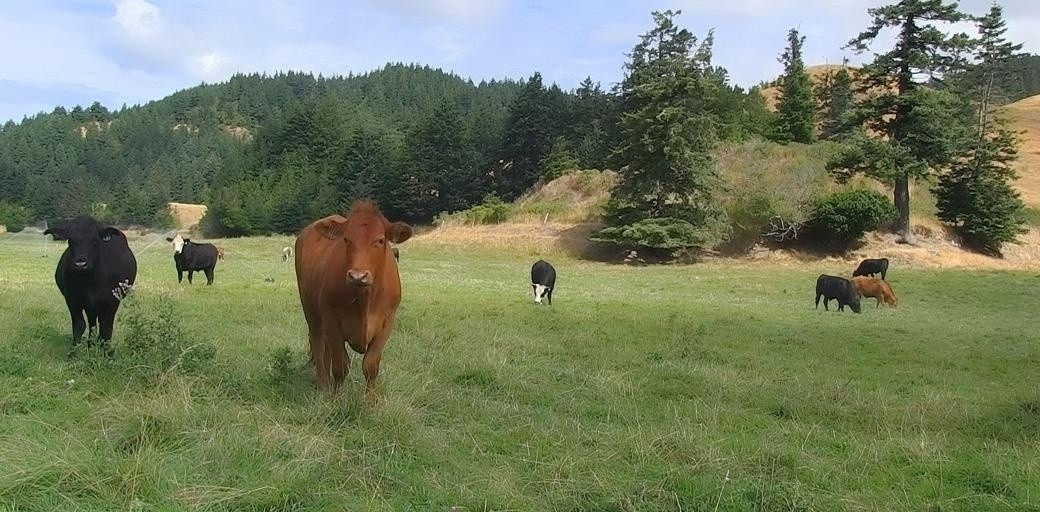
[281,246,293,262]
[165,234,218,286]
[815,273,863,314]
[852,257,889,280]
[851,276,899,309]
[294,198,413,406]
[43,215,137,360]
[530,260,556,307]
[217,247,225,263]
